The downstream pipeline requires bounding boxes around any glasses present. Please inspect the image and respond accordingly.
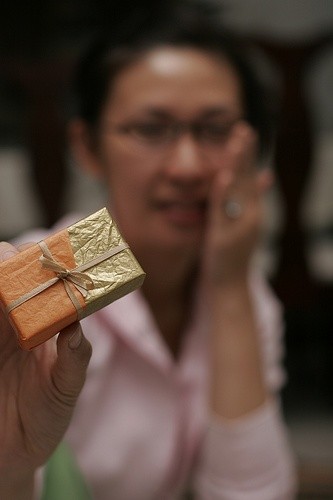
[119,106,235,148]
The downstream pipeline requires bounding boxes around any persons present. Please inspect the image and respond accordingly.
[0,24,297,500]
